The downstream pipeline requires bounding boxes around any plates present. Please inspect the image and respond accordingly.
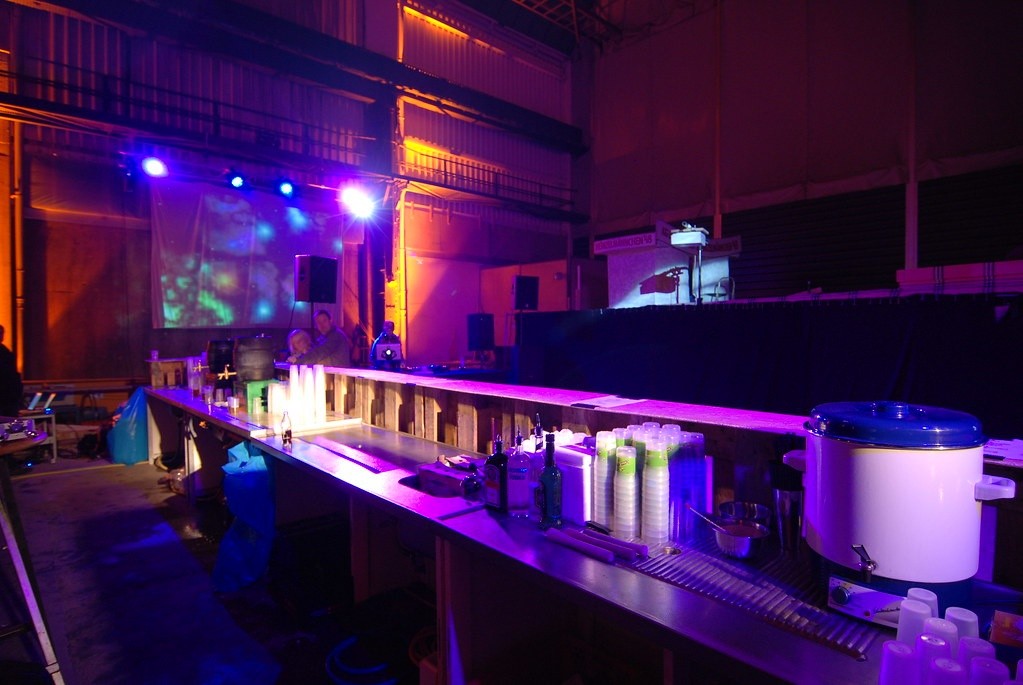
[0,422,23,430]
[0,433,28,441]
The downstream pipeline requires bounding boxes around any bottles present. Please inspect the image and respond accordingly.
[483,412,563,531]
[280,411,292,441]
[282,440,293,455]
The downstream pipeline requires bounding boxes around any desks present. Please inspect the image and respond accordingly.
[144,359,902,685]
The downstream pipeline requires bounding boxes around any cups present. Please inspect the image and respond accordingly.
[215,388,224,402]
[203,385,213,404]
[591,421,708,544]
[252,397,262,414]
[878,585,1023,685]
[227,397,239,414]
[288,364,327,427]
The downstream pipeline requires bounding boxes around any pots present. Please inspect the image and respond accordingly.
[782,396,1017,585]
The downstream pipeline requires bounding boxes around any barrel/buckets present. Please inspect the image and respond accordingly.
[206,335,274,380]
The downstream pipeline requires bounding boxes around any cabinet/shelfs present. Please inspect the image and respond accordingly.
[20,414,57,461]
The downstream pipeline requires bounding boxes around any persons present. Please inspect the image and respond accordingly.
[288,308,355,366]
[0,325,25,415]
[369,319,403,370]
[288,328,330,367]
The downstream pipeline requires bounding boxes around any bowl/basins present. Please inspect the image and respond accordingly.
[709,496,772,559]
